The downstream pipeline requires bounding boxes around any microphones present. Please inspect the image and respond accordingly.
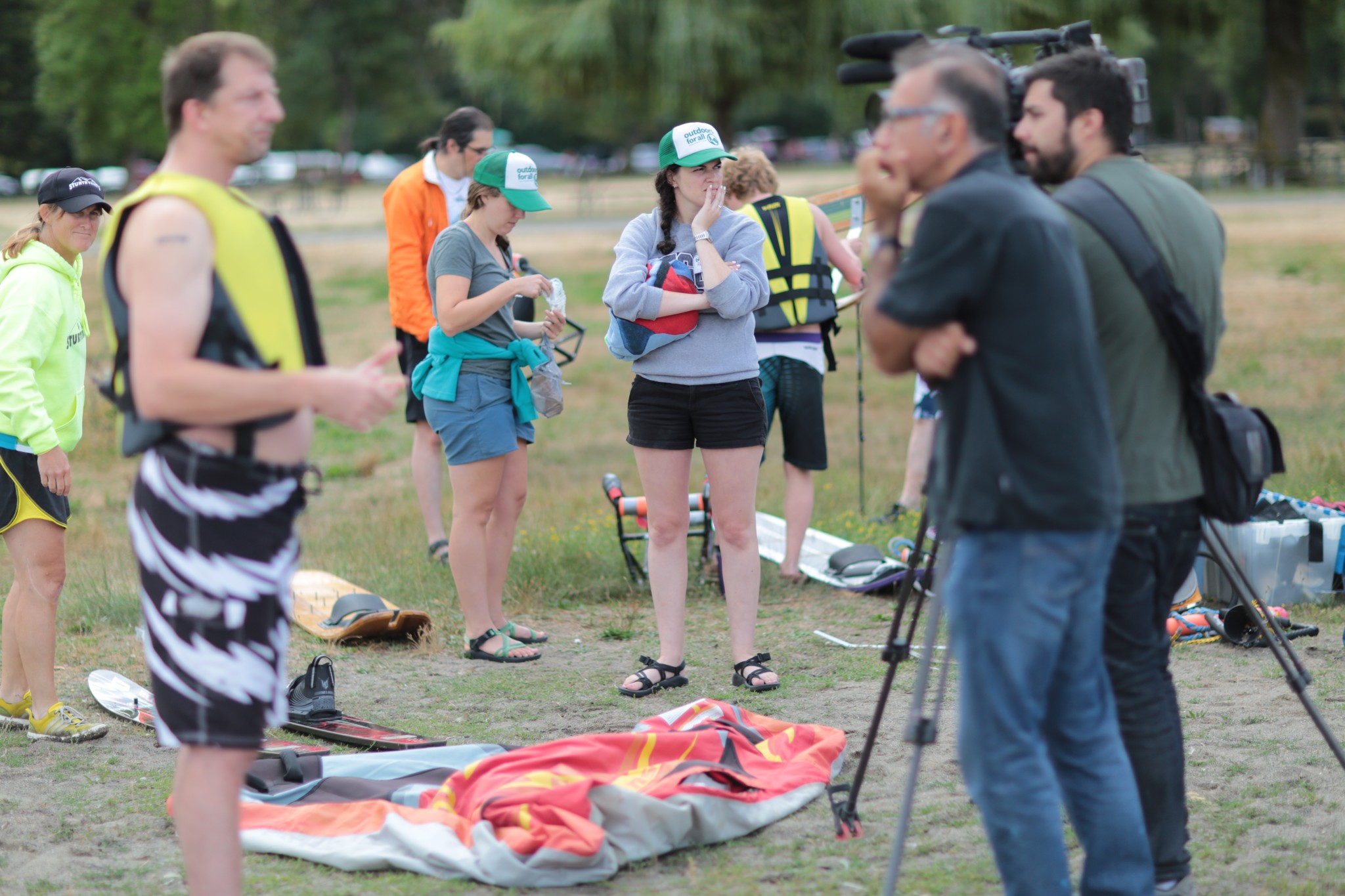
[836,31,925,83]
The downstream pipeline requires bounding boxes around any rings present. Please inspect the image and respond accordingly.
[718,205,722,209]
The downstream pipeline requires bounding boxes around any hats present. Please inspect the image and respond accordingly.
[473,150,554,212]
[658,122,739,169]
[38,166,114,217]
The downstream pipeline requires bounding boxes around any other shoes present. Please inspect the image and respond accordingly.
[1155,875,1193,896]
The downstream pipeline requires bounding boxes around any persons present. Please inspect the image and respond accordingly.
[1013,48,1228,896]
[410,150,567,662]
[872,370,941,526]
[384,105,494,565]
[861,42,1152,896]
[722,145,861,583]
[96,31,409,896]
[0,166,115,743]
[601,122,780,697]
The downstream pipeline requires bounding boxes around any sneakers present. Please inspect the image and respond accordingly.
[25,702,109,742]
[0,690,33,727]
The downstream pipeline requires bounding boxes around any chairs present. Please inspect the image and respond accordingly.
[603,471,715,587]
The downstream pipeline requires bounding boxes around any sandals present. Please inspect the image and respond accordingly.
[618,655,688,697]
[463,628,542,663]
[494,620,549,643]
[732,652,780,692]
[425,540,450,565]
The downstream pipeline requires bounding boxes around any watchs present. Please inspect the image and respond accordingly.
[868,233,901,250]
[693,229,713,244]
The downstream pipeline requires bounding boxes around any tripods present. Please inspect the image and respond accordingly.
[826,507,1345,896]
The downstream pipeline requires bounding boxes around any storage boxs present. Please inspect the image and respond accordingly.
[1203,489,1345,611]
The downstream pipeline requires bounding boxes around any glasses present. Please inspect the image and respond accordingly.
[462,143,497,156]
[879,104,948,124]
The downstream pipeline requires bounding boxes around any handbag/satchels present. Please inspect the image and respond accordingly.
[1186,386,1285,523]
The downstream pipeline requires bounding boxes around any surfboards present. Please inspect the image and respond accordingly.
[754,510,910,590]
[280,708,448,750]
[286,568,429,643]
[88,669,330,756]
